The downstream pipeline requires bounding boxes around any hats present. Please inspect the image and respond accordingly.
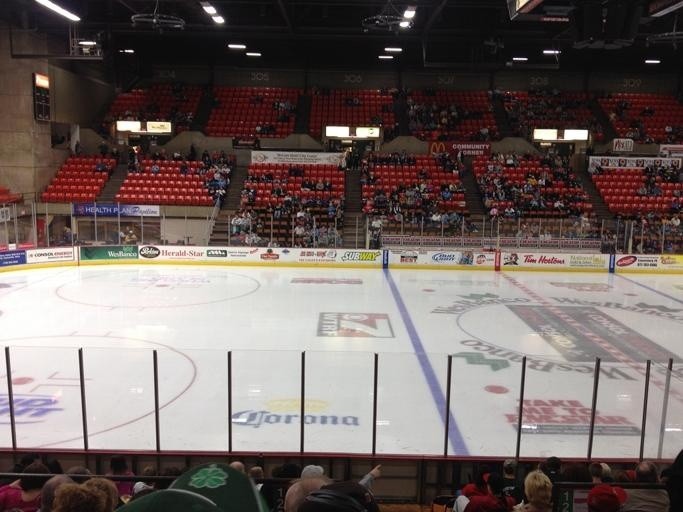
[587,484,627,508]
[301,465,323,478]
[114,462,269,512]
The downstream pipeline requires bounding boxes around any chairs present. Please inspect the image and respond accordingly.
[503,84,681,145]
[203,83,299,140]
[597,167,682,242]
[405,83,502,143]
[472,150,596,240]
[359,152,471,242]
[104,83,202,132]
[307,85,396,137]
[232,162,345,247]
[42,155,114,204]
[114,154,224,206]
